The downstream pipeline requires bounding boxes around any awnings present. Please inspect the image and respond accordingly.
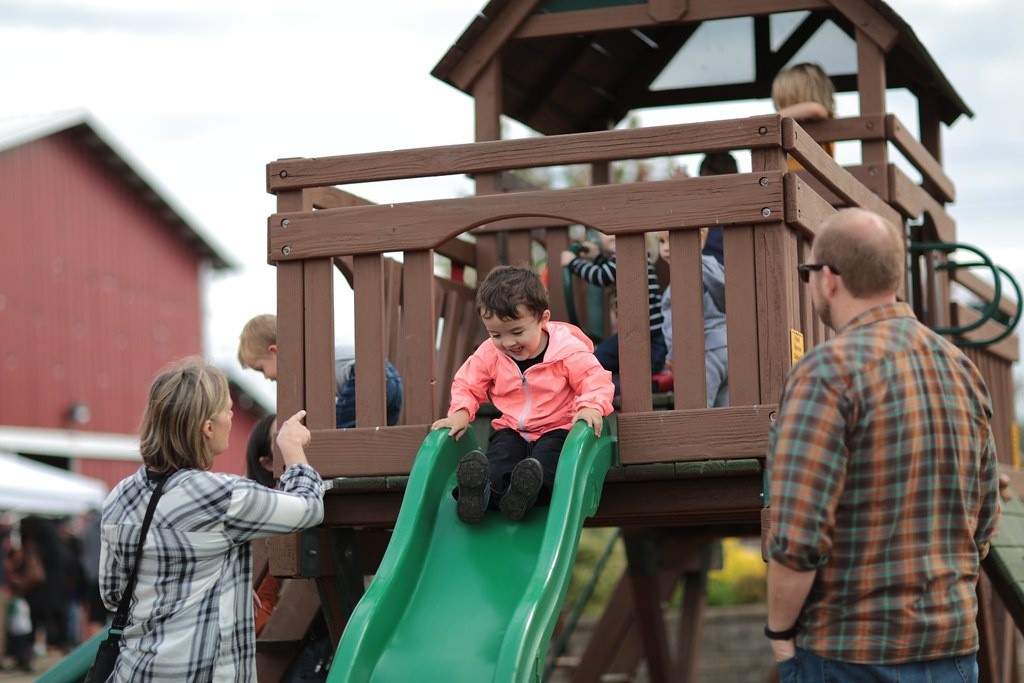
[2,449,107,522]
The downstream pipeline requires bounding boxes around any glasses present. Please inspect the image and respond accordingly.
[797,262,842,283]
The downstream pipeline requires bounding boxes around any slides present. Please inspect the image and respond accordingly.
[323,411,621,683]
[28,410,396,683]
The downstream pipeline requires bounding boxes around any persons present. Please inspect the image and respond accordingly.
[697,151,740,262]
[562,224,670,377]
[244,408,368,683]
[772,62,838,173]
[99,359,324,682]
[760,208,1003,683]
[656,228,731,408]
[236,316,404,427]
[428,265,615,525]
[1,512,90,678]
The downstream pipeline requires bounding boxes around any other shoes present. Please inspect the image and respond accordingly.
[498,457,544,521]
[651,372,674,393]
[456,449,492,524]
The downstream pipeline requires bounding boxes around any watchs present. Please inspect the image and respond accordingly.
[762,623,798,642]
